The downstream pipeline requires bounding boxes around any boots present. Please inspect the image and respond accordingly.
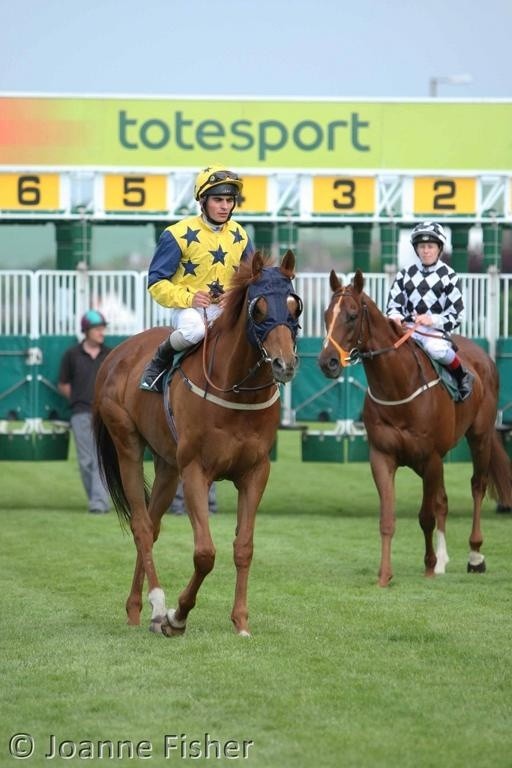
[445,352,475,402]
[138,335,183,392]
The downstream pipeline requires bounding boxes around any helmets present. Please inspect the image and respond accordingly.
[80,309,110,333]
[409,221,447,255]
[193,163,243,201]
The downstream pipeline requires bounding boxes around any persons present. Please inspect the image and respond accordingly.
[384,216,477,405]
[49,308,119,514]
[134,164,264,398]
[164,465,219,516]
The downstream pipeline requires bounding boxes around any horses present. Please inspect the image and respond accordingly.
[318,267,512,588]
[90,249,301,638]
